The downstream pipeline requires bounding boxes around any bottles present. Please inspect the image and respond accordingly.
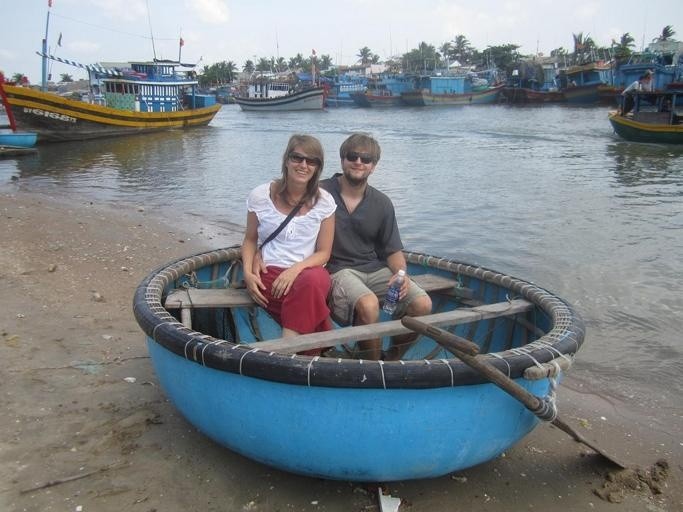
[383,269,405,316]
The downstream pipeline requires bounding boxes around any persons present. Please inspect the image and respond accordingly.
[250,131,432,361]
[620,74,651,119]
[242,133,338,359]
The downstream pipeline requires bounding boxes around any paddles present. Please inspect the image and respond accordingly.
[401,315,626,471]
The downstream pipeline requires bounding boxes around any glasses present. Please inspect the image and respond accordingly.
[343,151,374,164]
[286,152,317,166]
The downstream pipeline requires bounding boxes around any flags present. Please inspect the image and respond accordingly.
[180,39,184,46]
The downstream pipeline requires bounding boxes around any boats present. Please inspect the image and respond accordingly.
[291,71,372,104]
[400,67,508,105]
[230,47,331,112]
[0,58,224,144]
[500,34,683,106]
[132,242,588,484]
[0,133,38,149]
[348,71,430,106]
[608,81,683,145]
